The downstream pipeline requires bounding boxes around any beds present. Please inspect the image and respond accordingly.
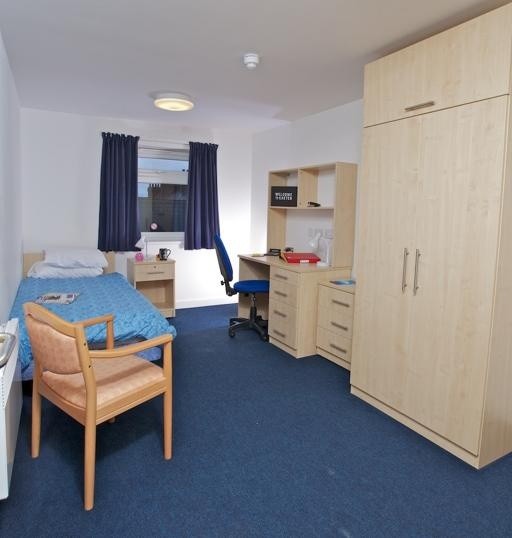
[7,249,175,382]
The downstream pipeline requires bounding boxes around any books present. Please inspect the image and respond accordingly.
[281,251,321,263]
[34,291,81,305]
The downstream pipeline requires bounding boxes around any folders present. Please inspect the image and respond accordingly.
[284,253,321,263]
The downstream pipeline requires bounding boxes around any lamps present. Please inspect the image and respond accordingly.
[153,91,195,112]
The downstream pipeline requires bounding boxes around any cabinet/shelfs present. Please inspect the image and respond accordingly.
[314,280,354,373]
[265,162,358,270]
[347,3,510,470]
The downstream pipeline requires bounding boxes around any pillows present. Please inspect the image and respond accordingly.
[26,248,109,279]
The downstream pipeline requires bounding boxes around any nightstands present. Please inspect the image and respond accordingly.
[125,257,177,319]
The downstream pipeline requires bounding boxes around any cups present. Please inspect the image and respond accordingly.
[159,248,171,261]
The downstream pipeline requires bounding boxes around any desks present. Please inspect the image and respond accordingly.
[236,253,351,361]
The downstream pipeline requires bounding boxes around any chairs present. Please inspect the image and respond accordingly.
[19,300,177,514]
[212,232,272,345]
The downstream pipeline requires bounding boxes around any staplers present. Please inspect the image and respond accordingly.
[307,201,321,207]
